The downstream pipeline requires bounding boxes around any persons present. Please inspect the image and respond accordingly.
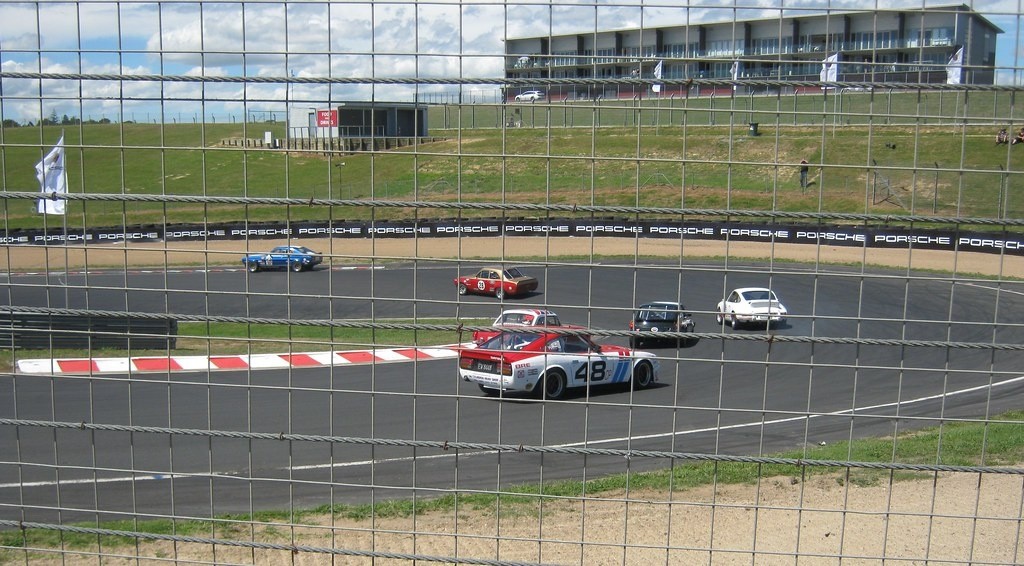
[800,158,809,187]
[1012,128,1024,144]
[996,129,1007,144]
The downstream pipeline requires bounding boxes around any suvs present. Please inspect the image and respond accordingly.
[515,90,544,101]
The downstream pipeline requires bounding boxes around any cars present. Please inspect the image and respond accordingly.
[472,309,589,349]
[629,301,696,348]
[453,265,536,299]
[716,288,788,329]
[242,245,322,272]
[458,332,662,400]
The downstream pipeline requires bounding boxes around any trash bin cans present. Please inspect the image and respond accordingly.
[748,122,759,136]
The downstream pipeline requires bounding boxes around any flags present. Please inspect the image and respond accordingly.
[730,58,739,90]
[820,53,837,90]
[651,60,662,92]
[946,47,963,85]
[35,137,68,216]
